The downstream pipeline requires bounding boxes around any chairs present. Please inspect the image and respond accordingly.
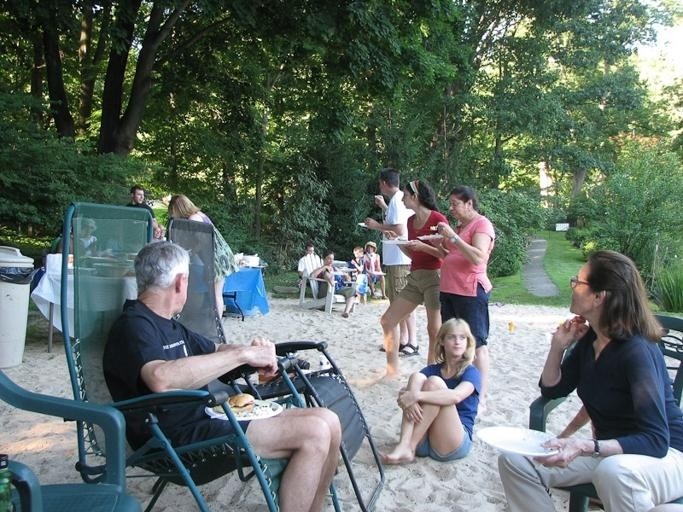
[528,318,683,512]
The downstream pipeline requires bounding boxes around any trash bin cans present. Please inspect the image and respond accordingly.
[0,246,34,369]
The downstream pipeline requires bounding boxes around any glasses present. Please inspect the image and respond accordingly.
[569,275,590,288]
[446,334,466,341]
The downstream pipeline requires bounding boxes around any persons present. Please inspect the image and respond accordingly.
[55,185,235,341]
[97,240,343,511]
[375,317,482,466]
[494,247,681,511]
[297,240,388,318]
[364,168,495,419]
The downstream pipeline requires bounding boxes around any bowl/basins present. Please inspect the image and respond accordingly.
[88,257,117,267]
[95,263,128,276]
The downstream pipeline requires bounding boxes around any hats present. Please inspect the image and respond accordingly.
[365,242,377,252]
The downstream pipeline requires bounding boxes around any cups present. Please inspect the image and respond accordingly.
[375,196,382,204]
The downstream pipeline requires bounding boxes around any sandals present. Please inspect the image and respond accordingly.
[379,342,419,358]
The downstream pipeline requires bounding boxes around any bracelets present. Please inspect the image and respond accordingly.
[449,233,458,245]
[589,437,600,460]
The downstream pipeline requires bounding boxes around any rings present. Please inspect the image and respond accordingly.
[440,227,445,233]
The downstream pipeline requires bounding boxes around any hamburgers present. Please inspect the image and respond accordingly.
[229,393,255,414]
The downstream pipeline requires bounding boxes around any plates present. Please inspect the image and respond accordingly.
[343,269,356,271]
[334,273,347,275]
[371,272,386,275]
[477,426,559,455]
[343,282,356,284]
[379,240,411,245]
[359,223,368,227]
[416,235,444,240]
[205,399,283,421]
